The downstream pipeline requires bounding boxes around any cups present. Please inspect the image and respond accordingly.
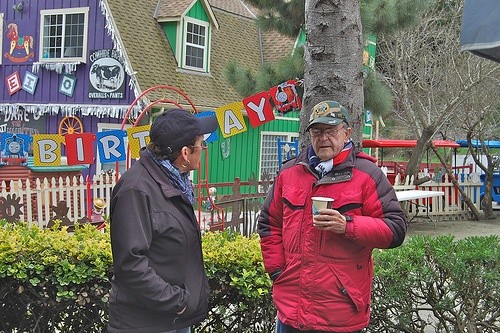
[311,197,334,226]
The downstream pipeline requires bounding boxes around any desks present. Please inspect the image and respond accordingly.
[396,190,444,230]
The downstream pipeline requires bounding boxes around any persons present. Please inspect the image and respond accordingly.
[107,108,209,333]
[258,100,406,333]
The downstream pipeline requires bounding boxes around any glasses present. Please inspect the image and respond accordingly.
[187,140,208,150]
[309,126,345,137]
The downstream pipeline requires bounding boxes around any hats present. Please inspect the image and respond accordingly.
[150,108,219,155]
[305,100,350,132]
